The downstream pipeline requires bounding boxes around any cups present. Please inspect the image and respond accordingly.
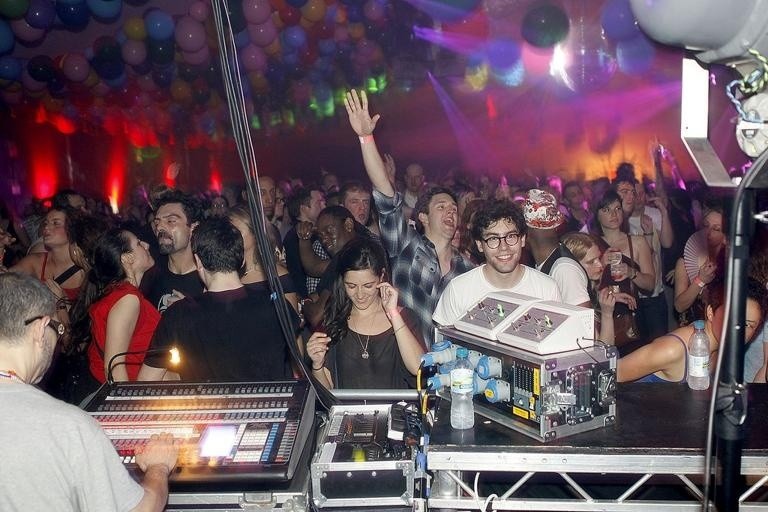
[610,249,622,280]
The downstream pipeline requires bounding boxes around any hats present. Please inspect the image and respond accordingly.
[520,189,565,229]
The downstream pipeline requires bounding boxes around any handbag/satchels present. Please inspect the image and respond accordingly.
[613,312,643,347]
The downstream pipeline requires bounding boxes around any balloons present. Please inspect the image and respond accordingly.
[1,0,434,136]
[410,0,656,95]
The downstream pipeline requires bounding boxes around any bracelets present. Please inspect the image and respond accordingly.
[359,130,374,144]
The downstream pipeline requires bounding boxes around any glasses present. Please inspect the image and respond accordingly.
[481,234,521,248]
[23,315,70,336]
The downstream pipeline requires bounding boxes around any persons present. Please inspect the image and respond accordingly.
[1,151,768,394]
[341,87,474,342]
[0,271,178,510]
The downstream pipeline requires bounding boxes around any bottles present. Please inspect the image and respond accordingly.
[451,347,475,430]
[687,321,711,393]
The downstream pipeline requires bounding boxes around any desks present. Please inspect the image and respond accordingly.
[425,382,768,512]
